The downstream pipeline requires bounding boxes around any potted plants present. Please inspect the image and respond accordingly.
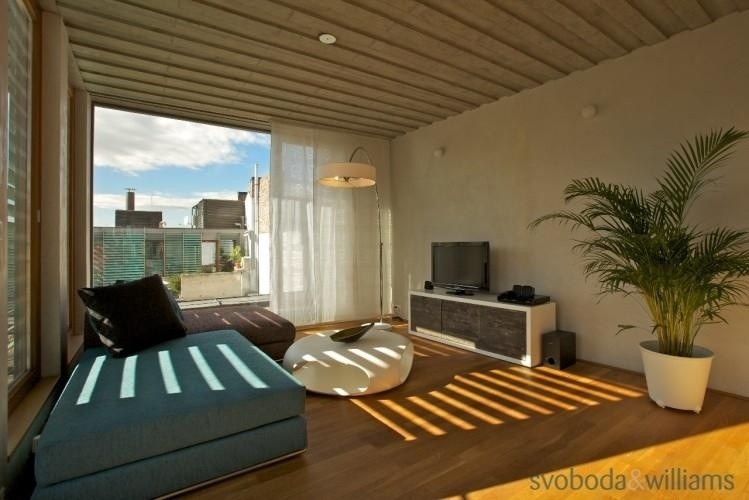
[527,125,749,415]
[230,245,242,268]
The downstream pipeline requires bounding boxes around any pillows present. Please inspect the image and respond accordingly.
[78,274,186,359]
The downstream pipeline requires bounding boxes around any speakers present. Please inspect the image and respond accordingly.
[542,331,577,370]
[424,280,434,294]
[513,284,535,299]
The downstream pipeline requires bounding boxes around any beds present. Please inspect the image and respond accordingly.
[281,328,414,398]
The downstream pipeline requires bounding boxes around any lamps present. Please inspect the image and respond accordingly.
[314,146,392,334]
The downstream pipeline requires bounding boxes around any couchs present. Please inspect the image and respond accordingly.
[84,278,296,362]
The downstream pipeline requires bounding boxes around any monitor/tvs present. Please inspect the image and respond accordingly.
[430,241,490,296]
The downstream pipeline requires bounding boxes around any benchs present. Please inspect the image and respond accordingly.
[31,328,308,500]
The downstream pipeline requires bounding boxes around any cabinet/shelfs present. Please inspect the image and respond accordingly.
[407,288,556,368]
